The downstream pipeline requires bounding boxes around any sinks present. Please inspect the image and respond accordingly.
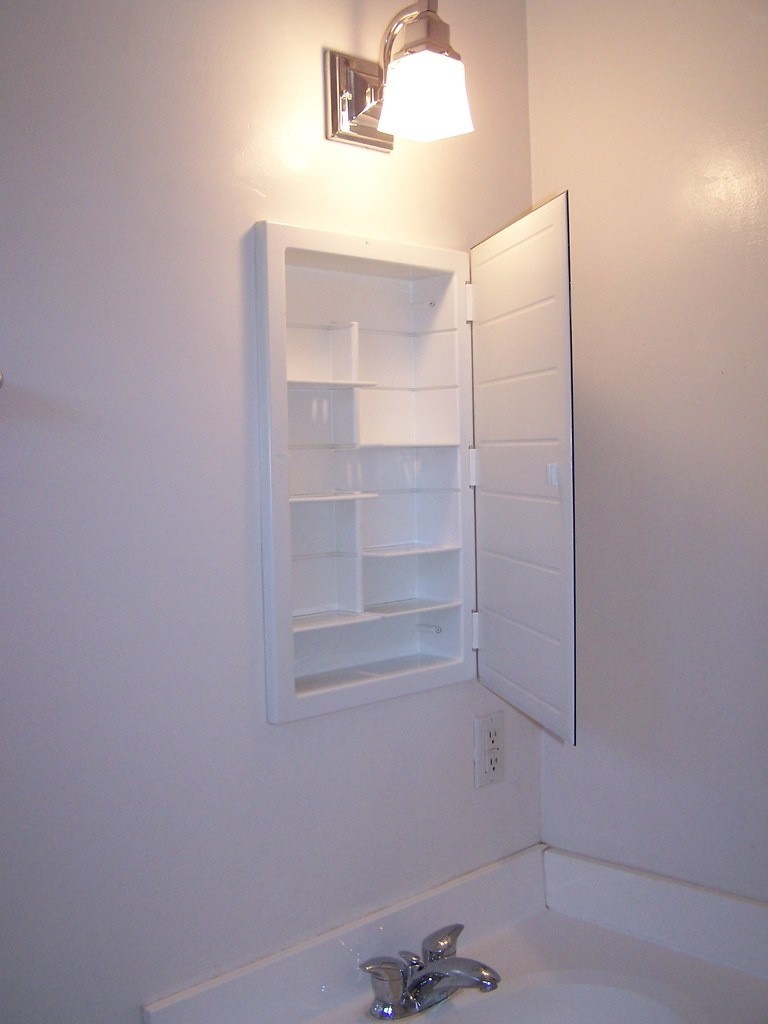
[430,981,678,1024]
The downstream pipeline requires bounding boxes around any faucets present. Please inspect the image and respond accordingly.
[357,924,502,1021]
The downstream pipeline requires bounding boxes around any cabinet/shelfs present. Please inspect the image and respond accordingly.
[253,190,575,748]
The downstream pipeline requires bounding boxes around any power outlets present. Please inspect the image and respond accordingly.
[473,710,506,790]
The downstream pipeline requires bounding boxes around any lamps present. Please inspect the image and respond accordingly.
[321,0,473,153]
